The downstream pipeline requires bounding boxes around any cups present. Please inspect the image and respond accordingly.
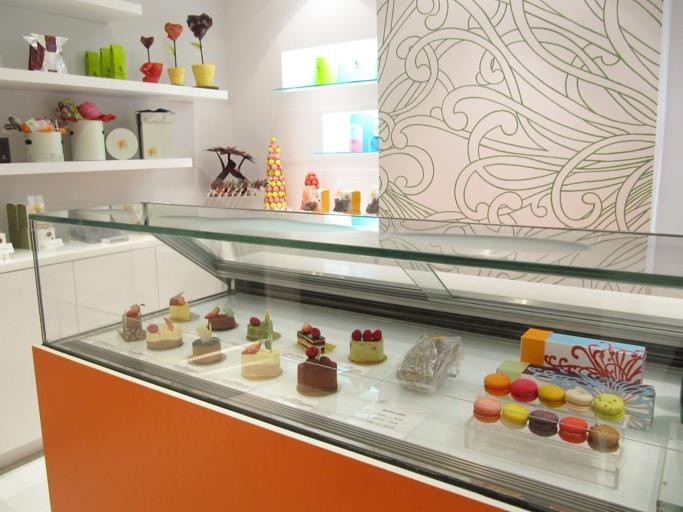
[67,119,106,161]
[22,131,64,164]
[71,206,109,226]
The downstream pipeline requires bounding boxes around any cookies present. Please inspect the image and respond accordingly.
[473,373,625,452]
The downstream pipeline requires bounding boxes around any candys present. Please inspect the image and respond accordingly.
[305,172,319,189]
[263,137,288,211]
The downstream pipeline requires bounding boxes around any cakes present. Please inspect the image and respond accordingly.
[119,294,387,397]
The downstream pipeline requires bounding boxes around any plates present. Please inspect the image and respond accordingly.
[110,201,145,228]
[103,126,139,161]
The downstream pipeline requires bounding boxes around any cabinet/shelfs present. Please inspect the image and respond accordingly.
[155,241,224,311]
[27,204,683,512]
[73,245,162,338]
[272,78,378,157]
[1,2,227,180]
[0,261,78,469]
